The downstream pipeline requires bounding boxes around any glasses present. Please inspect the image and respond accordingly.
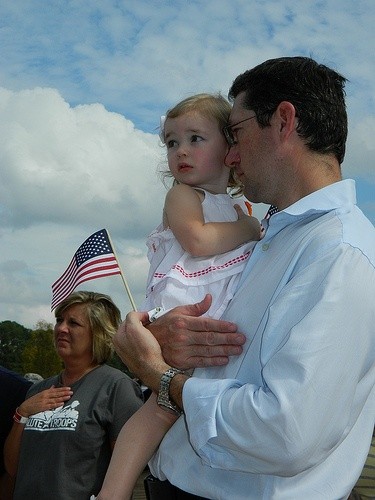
[223,109,275,145]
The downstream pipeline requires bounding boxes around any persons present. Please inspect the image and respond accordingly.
[0,366,35,471]
[12,290,148,500]
[93,96,266,499]
[110,56,375,500]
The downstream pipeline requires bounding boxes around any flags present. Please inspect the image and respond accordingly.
[36,228,125,313]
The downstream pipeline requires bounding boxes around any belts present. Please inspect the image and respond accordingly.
[144,474,208,500]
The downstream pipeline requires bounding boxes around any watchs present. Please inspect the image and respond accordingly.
[155,366,184,417]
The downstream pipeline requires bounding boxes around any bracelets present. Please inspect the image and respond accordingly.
[9,404,29,426]
